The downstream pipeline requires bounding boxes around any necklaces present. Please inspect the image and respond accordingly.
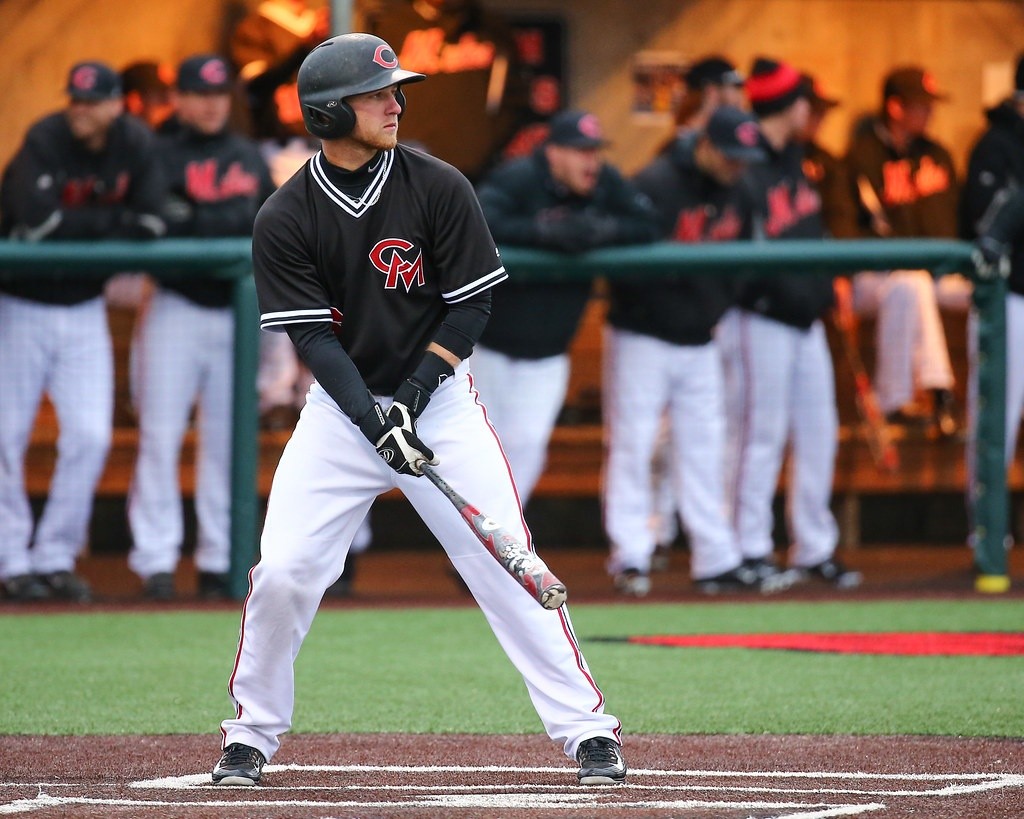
[341,148,405,218]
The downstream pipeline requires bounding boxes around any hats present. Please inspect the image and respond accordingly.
[175,52,237,94]
[802,68,839,118]
[742,52,809,118]
[685,55,746,92]
[879,63,953,102]
[550,105,612,150]
[704,105,771,164]
[65,60,125,101]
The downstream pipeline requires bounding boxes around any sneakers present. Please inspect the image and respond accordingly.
[211,745,266,788]
[575,737,629,785]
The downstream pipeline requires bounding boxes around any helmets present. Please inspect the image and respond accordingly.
[296,31,427,140]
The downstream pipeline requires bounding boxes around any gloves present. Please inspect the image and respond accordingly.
[358,403,441,478]
[383,379,436,438]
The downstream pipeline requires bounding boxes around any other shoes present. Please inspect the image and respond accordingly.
[1,565,92,603]
[691,558,801,596]
[195,567,232,598]
[807,555,864,592]
[141,569,183,601]
[884,390,960,437]
[612,567,653,598]
[327,551,353,604]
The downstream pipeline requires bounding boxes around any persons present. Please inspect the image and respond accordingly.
[127,53,379,604]
[832,62,963,445]
[961,54,1024,603]
[600,54,865,598]
[210,32,630,789]
[1,61,167,602]
[436,109,650,593]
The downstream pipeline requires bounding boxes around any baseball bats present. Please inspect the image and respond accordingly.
[416,459,568,610]
